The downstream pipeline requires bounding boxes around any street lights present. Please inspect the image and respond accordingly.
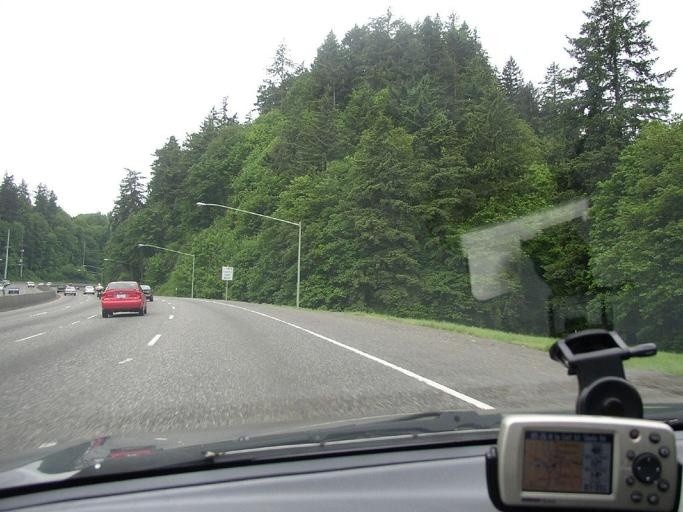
[137,243,193,299]
[104,258,142,282]
[195,202,301,306]
[82,265,103,282]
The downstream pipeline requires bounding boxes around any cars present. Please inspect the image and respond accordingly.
[0,279,153,318]
